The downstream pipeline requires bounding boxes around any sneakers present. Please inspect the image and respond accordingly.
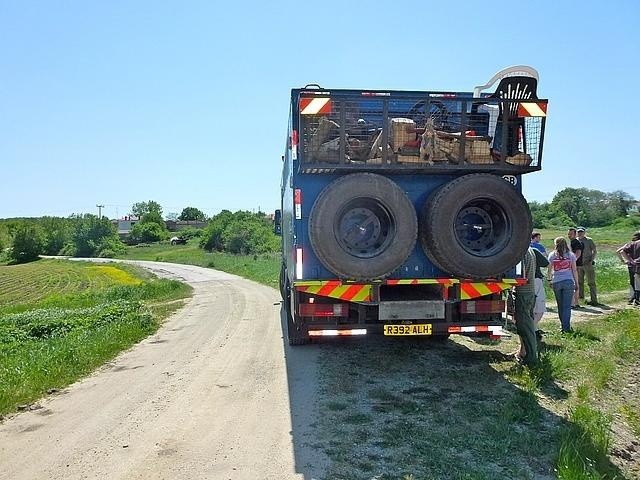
[587,300,597,305]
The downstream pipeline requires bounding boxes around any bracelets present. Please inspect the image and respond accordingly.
[546,278,553,281]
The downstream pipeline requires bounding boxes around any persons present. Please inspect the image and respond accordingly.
[546,237,580,335]
[567,228,583,310]
[615,231,640,305]
[530,247,551,337]
[530,232,548,260]
[575,226,599,306]
[505,246,539,371]
[619,238,640,307]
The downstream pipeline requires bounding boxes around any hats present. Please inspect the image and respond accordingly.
[577,227,585,232]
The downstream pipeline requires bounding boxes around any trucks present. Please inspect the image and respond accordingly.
[271,83,554,349]
